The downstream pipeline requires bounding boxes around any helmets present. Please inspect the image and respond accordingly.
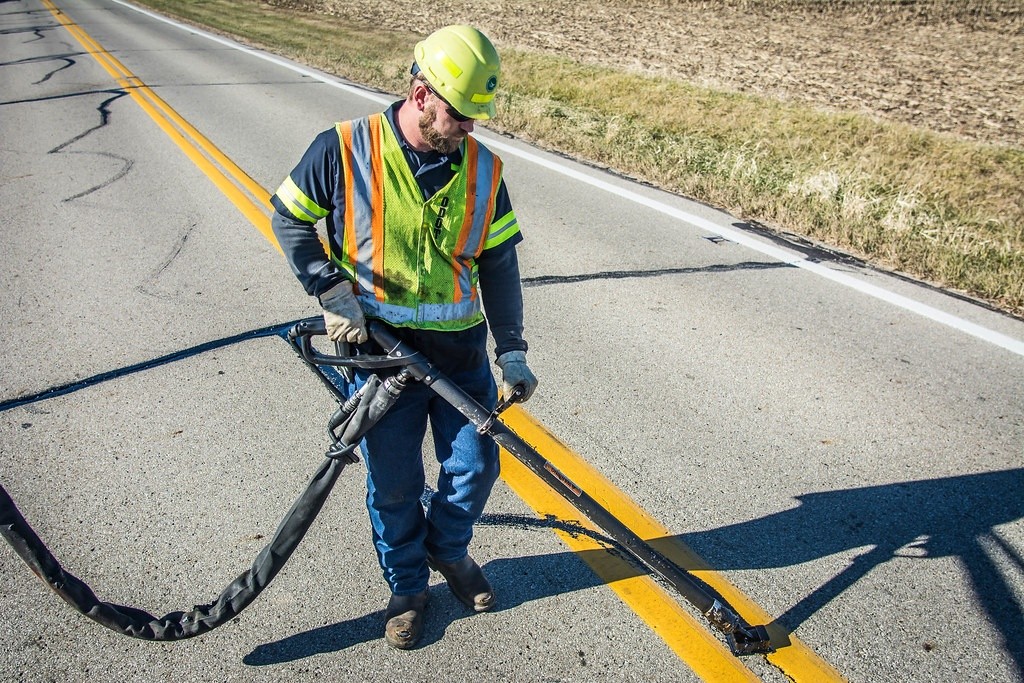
[417,24,501,120]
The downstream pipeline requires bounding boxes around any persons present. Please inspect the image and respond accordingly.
[269,26,538,648]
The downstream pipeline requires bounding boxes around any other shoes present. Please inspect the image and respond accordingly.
[426,548,497,612]
[383,582,432,650]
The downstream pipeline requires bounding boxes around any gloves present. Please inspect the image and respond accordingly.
[317,280,368,344]
[496,351,539,404]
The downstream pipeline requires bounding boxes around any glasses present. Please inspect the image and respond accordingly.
[424,84,474,122]
